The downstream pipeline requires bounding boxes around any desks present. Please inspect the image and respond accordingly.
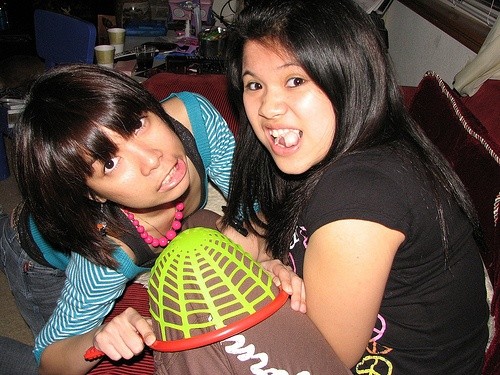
[114,28,180,83]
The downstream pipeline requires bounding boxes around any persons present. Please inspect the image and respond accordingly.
[0,63,306,375]
[151,0,490,375]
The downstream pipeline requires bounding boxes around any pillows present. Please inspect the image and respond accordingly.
[409,71,500,276]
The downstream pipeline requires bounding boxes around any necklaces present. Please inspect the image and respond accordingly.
[122,199,184,247]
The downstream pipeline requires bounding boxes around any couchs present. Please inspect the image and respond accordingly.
[84,71,500,375]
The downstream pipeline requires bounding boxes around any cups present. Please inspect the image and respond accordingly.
[94,45,114,69]
[0,101,12,179]
[201,37,218,57]
[107,28,126,55]
[135,45,156,69]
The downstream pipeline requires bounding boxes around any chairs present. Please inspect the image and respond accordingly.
[0,11,96,183]
